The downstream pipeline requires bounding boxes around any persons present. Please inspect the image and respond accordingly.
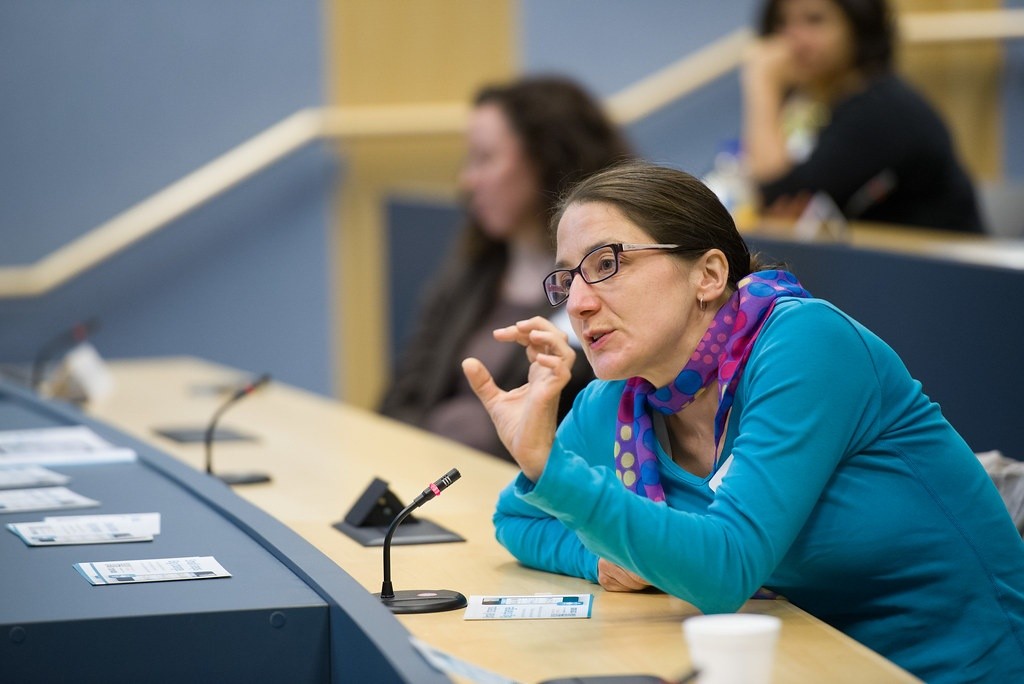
[462,165,1024,684]
[376,75,640,465]
[739,0,986,235]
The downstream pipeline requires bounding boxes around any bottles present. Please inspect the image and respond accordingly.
[700,139,762,237]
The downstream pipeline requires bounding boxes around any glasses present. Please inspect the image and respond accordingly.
[541,243,682,309]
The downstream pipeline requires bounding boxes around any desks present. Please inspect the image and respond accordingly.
[382,196,1024,462]
[8,353,925,684]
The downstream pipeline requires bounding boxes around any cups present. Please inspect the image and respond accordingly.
[682,613,779,684]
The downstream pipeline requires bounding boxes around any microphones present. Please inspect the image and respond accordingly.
[29,317,103,392]
[371,468,468,615]
[203,371,271,484]
